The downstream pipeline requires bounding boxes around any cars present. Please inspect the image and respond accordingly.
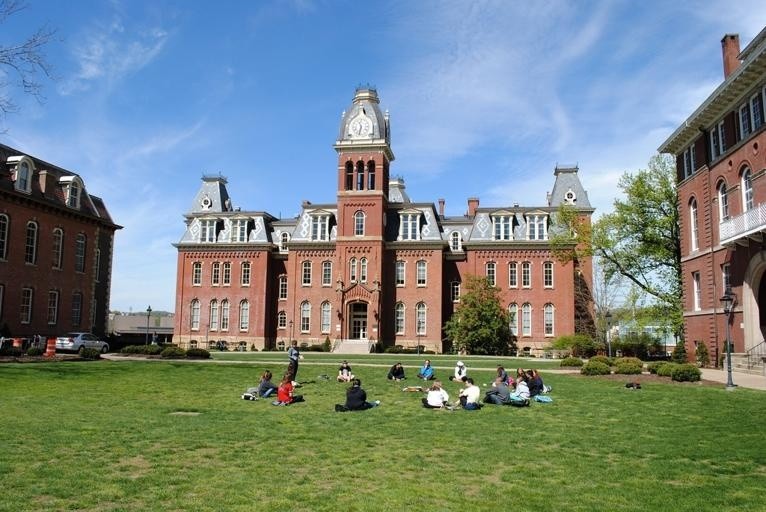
[55,331,110,355]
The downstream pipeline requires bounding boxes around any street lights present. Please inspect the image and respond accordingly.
[719,292,734,387]
[604,311,612,357]
[145,305,152,344]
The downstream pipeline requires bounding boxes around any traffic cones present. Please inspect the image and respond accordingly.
[43,339,56,357]
[12,337,23,352]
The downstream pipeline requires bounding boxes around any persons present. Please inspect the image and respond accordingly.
[483,379,511,406]
[511,367,543,407]
[258,370,277,399]
[337,360,355,382]
[417,359,434,382]
[287,339,304,382]
[277,373,303,406]
[455,378,480,409]
[493,363,510,387]
[345,379,381,411]
[421,379,450,409]
[449,360,467,383]
[388,362,405,382]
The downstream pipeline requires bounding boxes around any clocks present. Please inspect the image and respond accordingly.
[350,117,370,137]
